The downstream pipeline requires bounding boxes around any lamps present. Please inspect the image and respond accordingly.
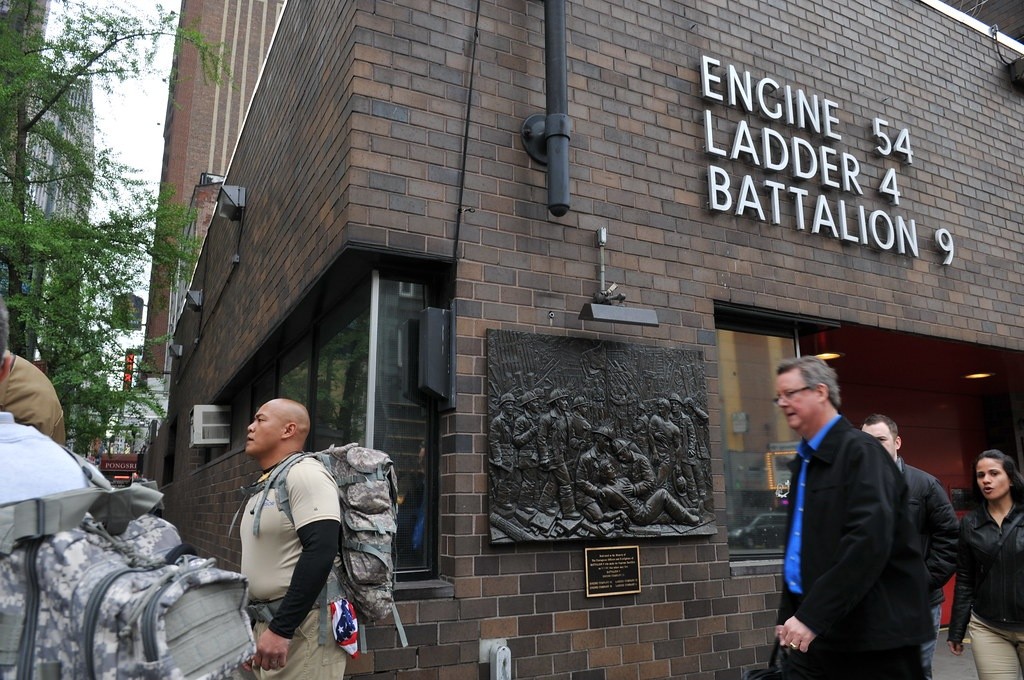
[217,185,245,222]
[185,291,203,311]
[169,345,183,360]
[578,226,659,327]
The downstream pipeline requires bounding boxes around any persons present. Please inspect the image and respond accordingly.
[861,412,960,680]
[239,398,356,680]
[397,446,426,568]
[947,449,1024,680]
[769,355,936,679]
[0,293,103,506]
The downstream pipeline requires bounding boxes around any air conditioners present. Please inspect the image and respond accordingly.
[188,404,230,449]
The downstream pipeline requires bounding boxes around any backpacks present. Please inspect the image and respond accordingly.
[0,444,260,680]
[227,442,409,655]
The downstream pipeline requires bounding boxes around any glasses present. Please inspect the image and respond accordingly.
[772,387,810,405]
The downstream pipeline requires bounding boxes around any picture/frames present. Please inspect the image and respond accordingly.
[948,483,976,515]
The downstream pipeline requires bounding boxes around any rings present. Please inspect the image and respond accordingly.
[789,642,797,648]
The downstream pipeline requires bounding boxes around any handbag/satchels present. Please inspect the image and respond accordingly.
[741,633,788,680]
